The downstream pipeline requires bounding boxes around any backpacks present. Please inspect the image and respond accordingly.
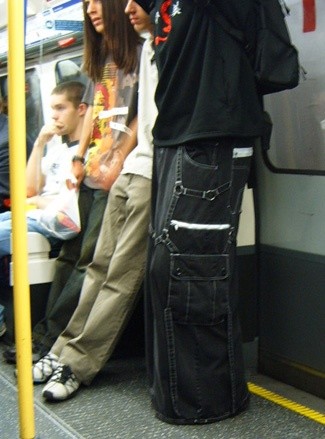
[203,0,306,99]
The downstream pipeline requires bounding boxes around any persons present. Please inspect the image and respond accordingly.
[0,93,34,336]
[0,82,87,334]
[149,0,300,425]
[15,1,159,400]
[4,1,146,362]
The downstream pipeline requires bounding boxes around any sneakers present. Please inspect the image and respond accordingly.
[42,363,82,400]
[14,352,59,383]
[2,339,50,363]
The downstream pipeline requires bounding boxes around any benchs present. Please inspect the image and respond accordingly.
[9,231,57,286]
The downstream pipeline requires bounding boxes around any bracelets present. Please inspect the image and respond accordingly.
[72,156,85,165]
